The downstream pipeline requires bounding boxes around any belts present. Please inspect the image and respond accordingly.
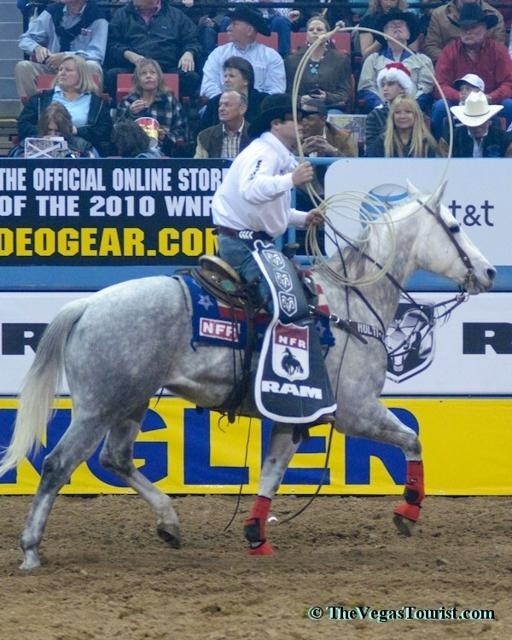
[211,226,271,242]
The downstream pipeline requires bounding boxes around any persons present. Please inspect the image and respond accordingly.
[8,0,512,158]
[208,92,337,424]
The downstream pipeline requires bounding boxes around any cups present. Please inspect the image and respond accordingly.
[134,115,159,151]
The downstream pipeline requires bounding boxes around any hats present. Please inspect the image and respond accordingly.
[375,61,414,97]
[450,91,503,129]
[371,7,420,47]
[225,5,271,37]
[247,92,320,134]
[446,2,498,31]
[452,73,485,92]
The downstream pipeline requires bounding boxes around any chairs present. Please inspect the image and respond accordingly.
[116,73,180,106]
[38,74,99,96]
[289,31,355,105]
[216,32,279,52]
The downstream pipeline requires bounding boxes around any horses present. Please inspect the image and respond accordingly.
[0,176,497,573]
